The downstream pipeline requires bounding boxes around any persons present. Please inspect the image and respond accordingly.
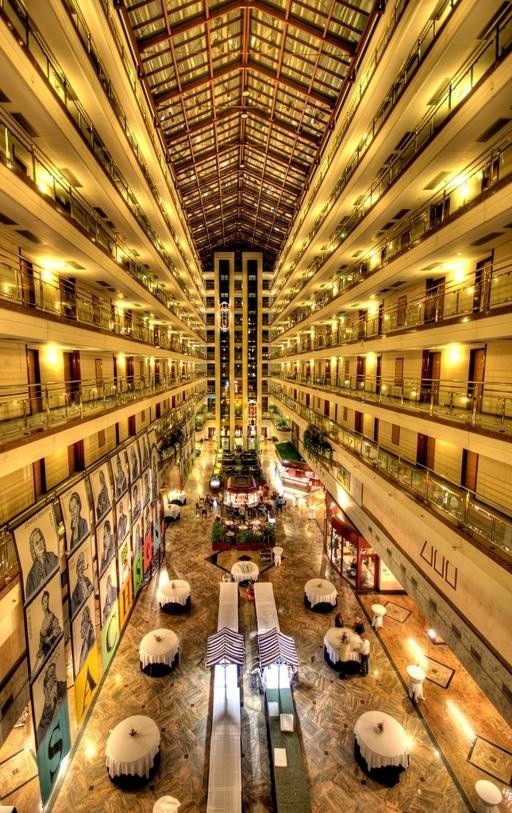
[22,526,59,597]
[335,611,345,627]
[262,486,270,497]
[234,446,242,453]
[238,503,245,524]
[70,551,94,615]
[352,616,364,635]
[97,436,151,627]
[78,605,95,668]
[242,502,248,509]
[275,496,283,505]
[206,493,213,504]
[67,491,90,549]
[36,590,62,658]
[35,663,68,746]
